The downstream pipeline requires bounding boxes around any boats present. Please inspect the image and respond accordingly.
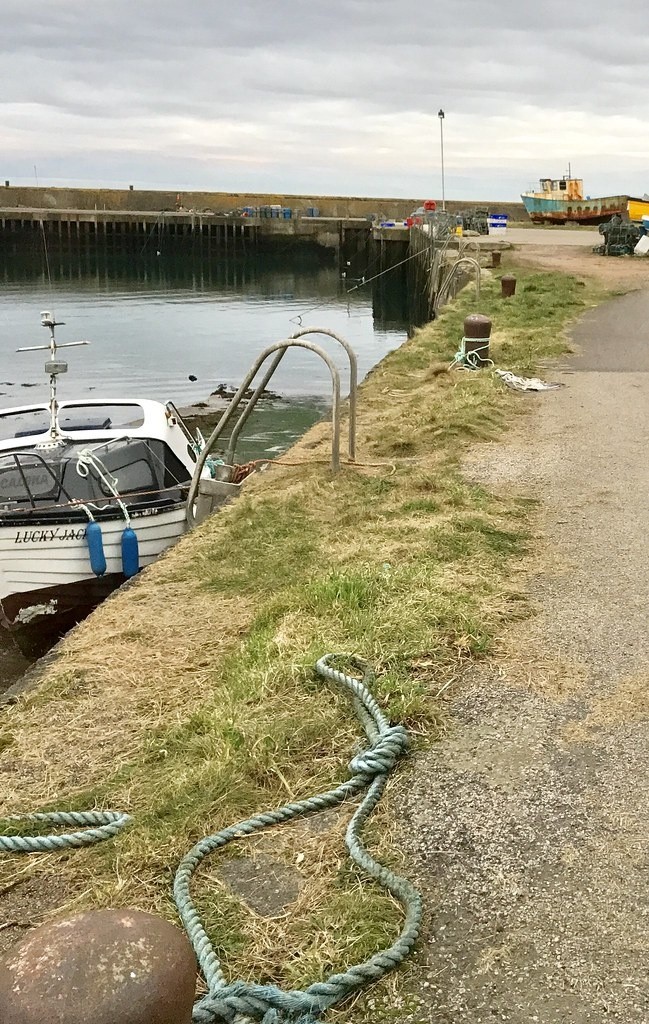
[520,162,628,222]
[0,310,220,662]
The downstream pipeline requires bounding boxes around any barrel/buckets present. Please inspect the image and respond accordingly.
[380,221,416,226]
[216,204,320,219]
[485,212,506,234]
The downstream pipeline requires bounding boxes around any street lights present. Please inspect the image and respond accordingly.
[438,109,447,213]
